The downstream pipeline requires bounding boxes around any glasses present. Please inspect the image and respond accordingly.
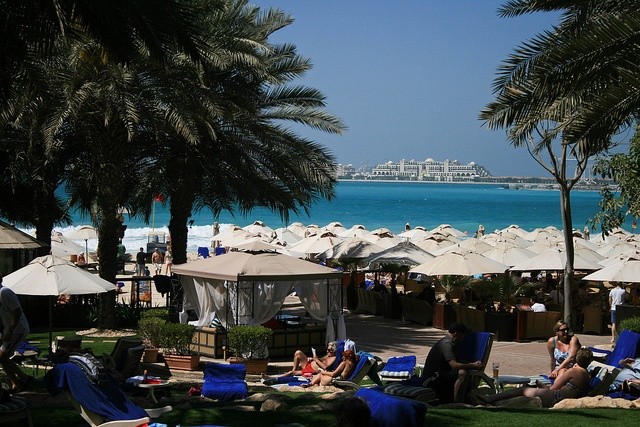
[326,349,333,352]
[558,328,569,332]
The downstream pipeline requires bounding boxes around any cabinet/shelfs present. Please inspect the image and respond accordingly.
[384,294,403,319]
[433,303,456,330]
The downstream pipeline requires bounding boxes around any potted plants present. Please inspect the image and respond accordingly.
[142,318,166,363]
[152,324,200,372]
[226,324,274,375]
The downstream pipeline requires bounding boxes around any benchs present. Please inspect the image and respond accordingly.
[454,306,486,332]
[403,296,432,326]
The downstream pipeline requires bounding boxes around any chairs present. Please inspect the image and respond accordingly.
[202,361,248,397]
[272,353,376,392]
[62,353,225,427]
[53,336,82,364]
[128,346,145,362]
[95,337,143,361]
[384,332,494,402]
[526,312,561,337]
[344,338,382,361]
[606,328,639,367]
[378,355,425,382]
[261,340,344,387]
[356,388,426,427]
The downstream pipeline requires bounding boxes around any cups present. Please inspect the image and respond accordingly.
[493,363,499,379]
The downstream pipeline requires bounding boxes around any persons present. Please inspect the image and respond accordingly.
[164,246,173,277]
[544,287,564,304]
[136,248,147,275]
[77,252,85,263]
[300,350,355,388]
[143,266,150,277]
[421,322,483,405]
[510,278,535,298]
[429,287,441,306]
[152,248,163,275]
[546,320,581,385]
[529,296,547,312]
[260,341,339,380]
[577,283,591,300]
[476,302,486,311]
[497,303,506,313]
[332,398,380,427]
[542,274,556,292]
[371,280,389,293]
[608,282,626,343]
[486,298,496,312]
[0,272,33,391]
[416,287,430,301]
[474,347,594,407]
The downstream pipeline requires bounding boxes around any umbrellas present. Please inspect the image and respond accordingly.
[3,251,119,359]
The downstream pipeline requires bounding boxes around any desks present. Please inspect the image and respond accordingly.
[126,378,169,403]
[14,350,38,378]
[492,376,530,394]
